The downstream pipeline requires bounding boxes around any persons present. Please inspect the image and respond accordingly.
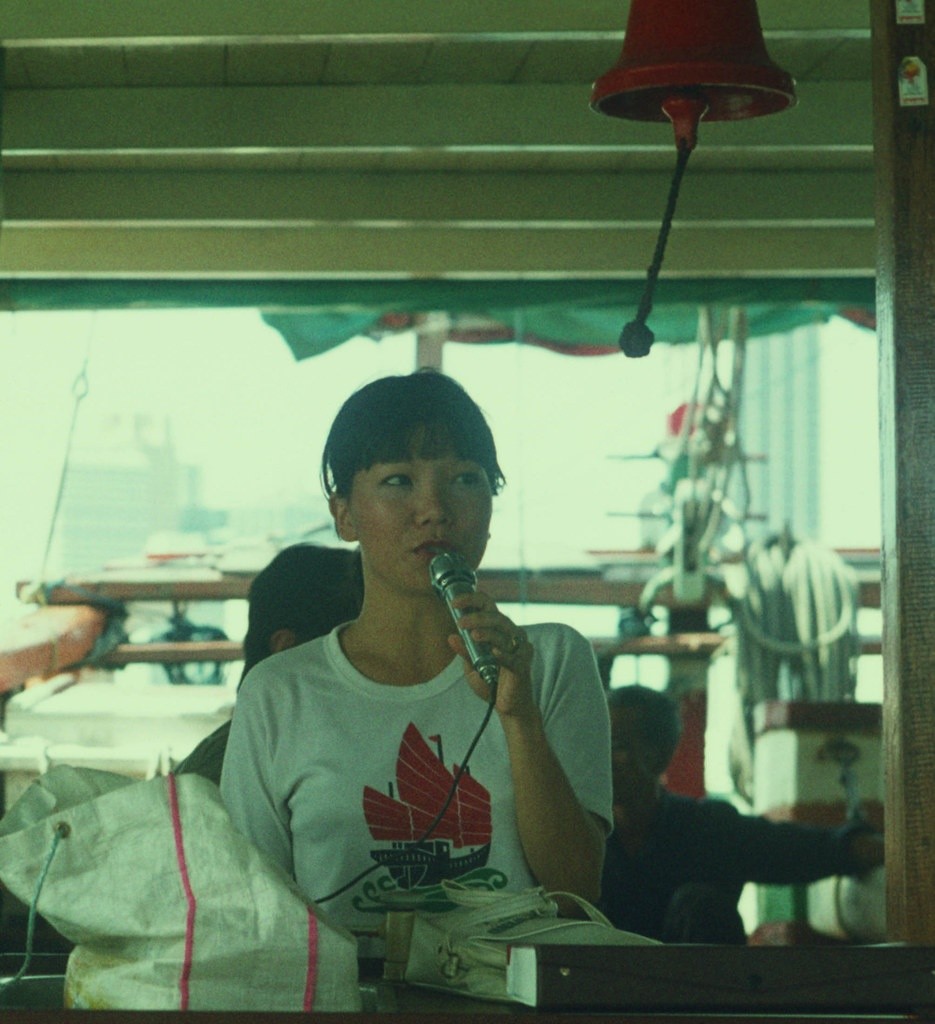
[595,685,884,948]
[173,544,365,786]
[217,365,613,962]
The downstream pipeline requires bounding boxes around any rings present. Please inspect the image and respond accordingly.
[507,632,524,656]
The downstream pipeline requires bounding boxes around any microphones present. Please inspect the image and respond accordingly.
[427,551,500,685]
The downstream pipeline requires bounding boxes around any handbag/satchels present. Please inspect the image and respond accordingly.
[403,877,665,1003]
[0,755,363,1014]
